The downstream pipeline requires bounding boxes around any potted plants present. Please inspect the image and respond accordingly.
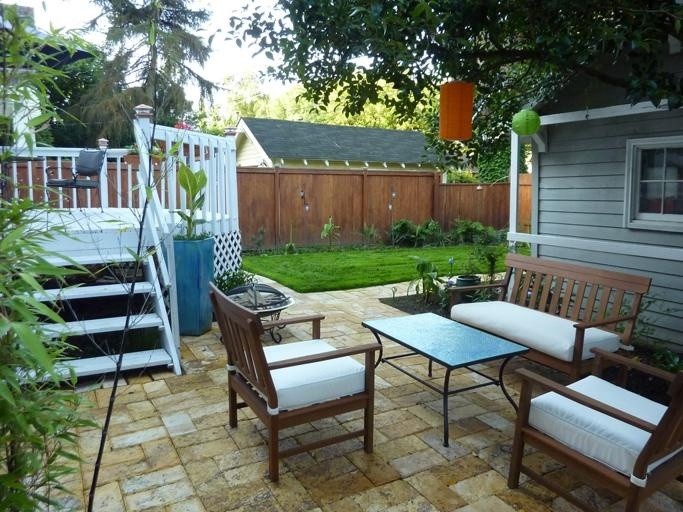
[160,159,216,337]
[456,246,481,302]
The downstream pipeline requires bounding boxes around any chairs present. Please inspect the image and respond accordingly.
[207,280,381,483]
[507,347,682,511]
[46,147,105,215]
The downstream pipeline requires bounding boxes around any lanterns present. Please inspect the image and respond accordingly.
[512,109,542,136]
[437,76,474,142]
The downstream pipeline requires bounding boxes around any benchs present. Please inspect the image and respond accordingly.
[447,253,652,390]
